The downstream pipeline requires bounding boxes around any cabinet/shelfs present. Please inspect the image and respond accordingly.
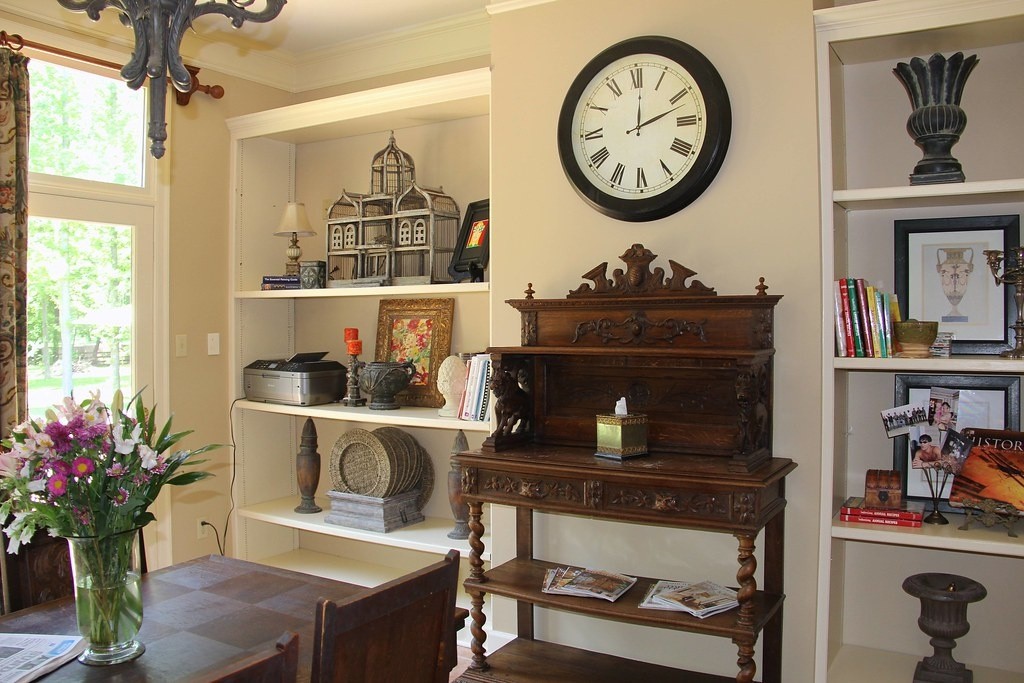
[813,0,1024,683]
[225,65,492,650]
[453,242,797,683]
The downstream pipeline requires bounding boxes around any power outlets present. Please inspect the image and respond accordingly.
[197,517,209,539]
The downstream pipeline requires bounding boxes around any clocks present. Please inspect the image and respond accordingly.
[557,37,733,224]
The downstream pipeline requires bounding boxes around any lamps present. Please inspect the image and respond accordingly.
[271,201,316,273]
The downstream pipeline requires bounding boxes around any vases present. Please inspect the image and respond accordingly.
[893,52,980,184]
[900,573,988,683]
[63,524,145,666]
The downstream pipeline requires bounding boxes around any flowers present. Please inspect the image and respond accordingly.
[0,381,237,557]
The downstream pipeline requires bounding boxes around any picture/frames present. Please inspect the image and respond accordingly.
[374,298,454,409]
[452,197,489,270]
[893,214,1021,354]
[893,372,1023,517]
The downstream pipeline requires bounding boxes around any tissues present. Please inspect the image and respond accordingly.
[596,397,650,456]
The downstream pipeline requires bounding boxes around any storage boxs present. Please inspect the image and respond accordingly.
[864,469,904,510]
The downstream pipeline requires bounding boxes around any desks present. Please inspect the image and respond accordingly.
[0,552,468,682]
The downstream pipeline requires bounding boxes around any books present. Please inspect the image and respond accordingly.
[834,278,903,358]
[839,496,926,527]
[929,331,954,358]
[561,566,637,602]
[638,581,696,611]
[458,355,490,423]
[653,579,739,619]
[541,565,608,597]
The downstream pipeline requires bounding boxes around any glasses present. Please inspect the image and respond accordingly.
[920,441,927,444]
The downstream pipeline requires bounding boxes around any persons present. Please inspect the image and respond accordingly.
[933,398,953,431]
[884,407,927,431]
[910,434,941,468]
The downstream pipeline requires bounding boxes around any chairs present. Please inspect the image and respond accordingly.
[309,549,460,682]
[200,631,299,683]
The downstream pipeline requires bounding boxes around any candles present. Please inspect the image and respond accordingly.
[347,340,363,354]
[343,327,358,344]
